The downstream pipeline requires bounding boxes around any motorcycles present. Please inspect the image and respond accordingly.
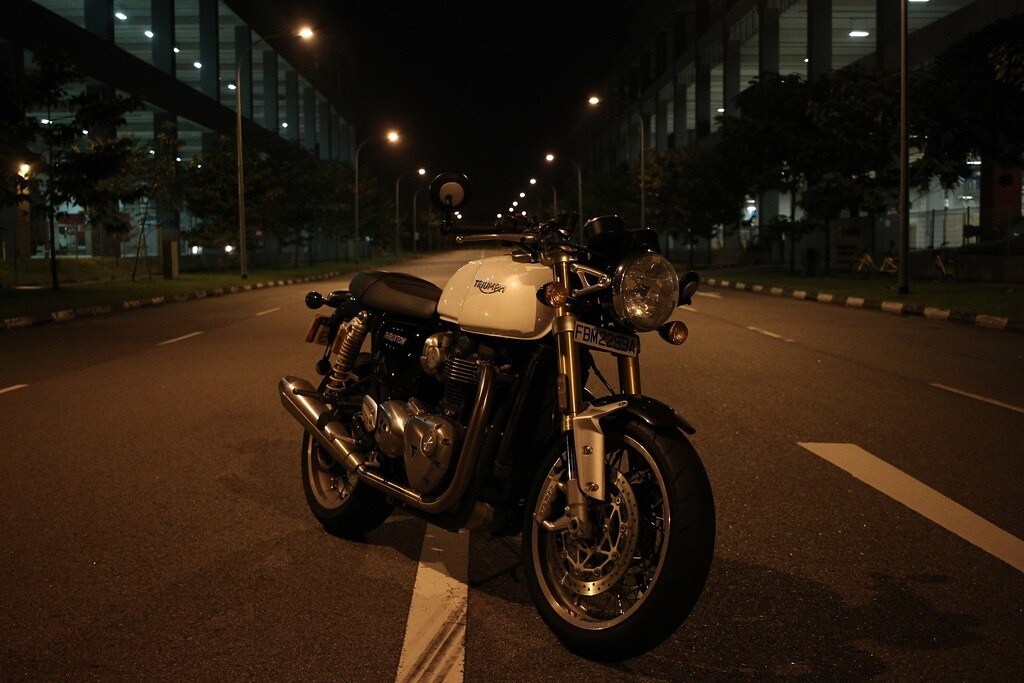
[272,211,716,662]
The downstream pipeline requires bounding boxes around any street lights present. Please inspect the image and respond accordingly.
[393,164,426,260]
[412,181,465,253]
[495,189,535,230]
[356,126,401,263]
[587,93,647,228]
[234,22,320,280]
[543,153,584,246]
[529,177,558,218]
